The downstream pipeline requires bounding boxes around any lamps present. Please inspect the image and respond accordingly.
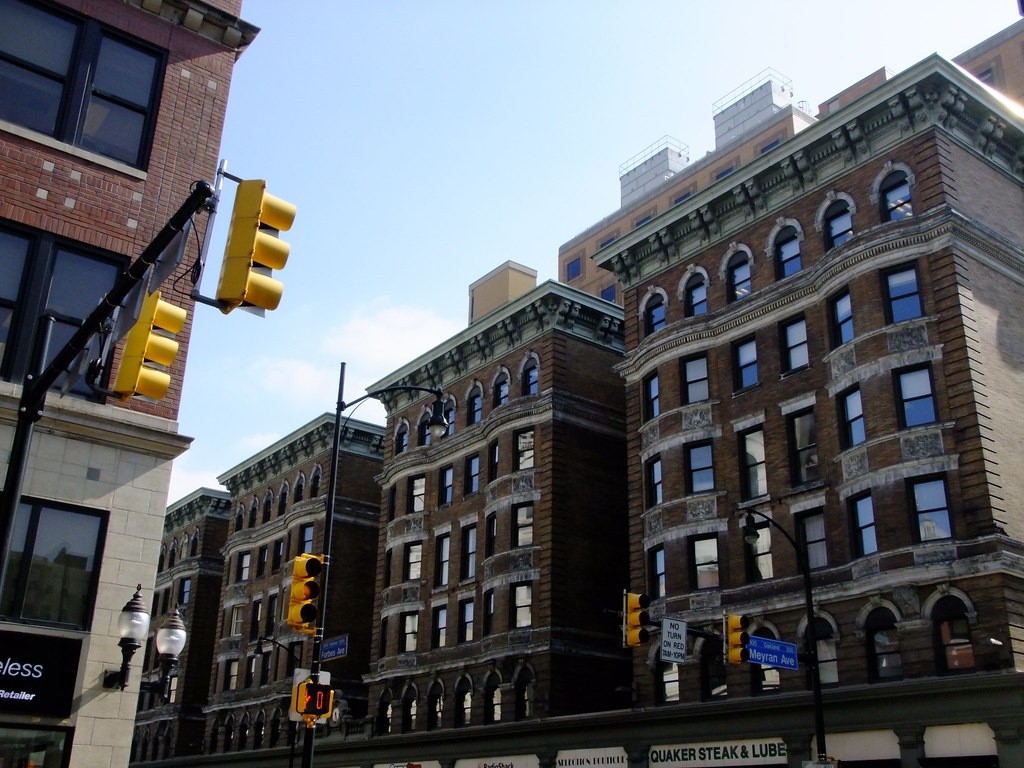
[140,603,187,705]
[103,584,150,692]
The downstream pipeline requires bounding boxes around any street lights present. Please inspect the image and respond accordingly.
[745,506,828,764]
[252,635,301,768]
[301,383,448,767]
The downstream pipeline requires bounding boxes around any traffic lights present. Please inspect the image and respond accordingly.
[215,180,297,316]
[286,552,322,628]
[728,614,750,664]
[113,289,188,401]
[293,682,335,722]
[627,592,650,649]
